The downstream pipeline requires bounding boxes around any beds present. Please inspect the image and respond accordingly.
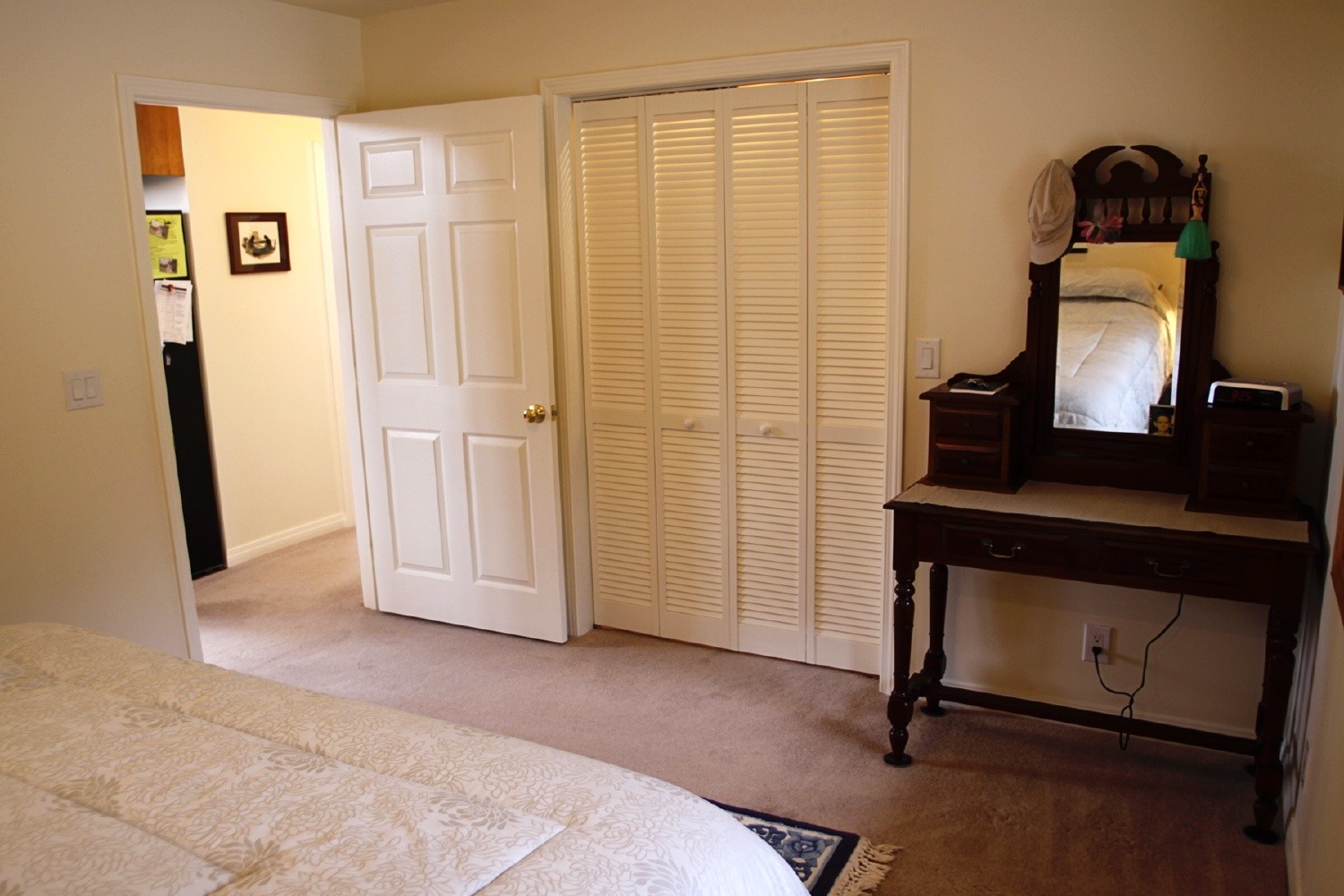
[1054,268,1177,432]
[0,624,816,896]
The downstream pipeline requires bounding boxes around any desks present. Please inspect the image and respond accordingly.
[882,484,1330,841]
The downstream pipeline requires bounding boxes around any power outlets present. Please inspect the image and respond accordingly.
[1081,622,1112,666]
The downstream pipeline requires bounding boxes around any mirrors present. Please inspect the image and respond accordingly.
[1023,142,1221,485]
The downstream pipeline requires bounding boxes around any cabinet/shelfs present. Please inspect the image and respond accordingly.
[916,371,1025,494]
[1192,373,1314,515]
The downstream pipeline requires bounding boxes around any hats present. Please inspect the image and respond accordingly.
[1027,159,1076,265]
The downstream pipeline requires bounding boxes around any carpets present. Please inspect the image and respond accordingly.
[701,795,907,896]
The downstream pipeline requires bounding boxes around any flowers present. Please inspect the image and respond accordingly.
[1078,211,1123,247]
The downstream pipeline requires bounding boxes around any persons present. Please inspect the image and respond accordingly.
[1151,413,1172,436]
[1175,173,1212,260]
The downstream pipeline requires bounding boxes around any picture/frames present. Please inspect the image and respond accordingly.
[226,210,293,276]
